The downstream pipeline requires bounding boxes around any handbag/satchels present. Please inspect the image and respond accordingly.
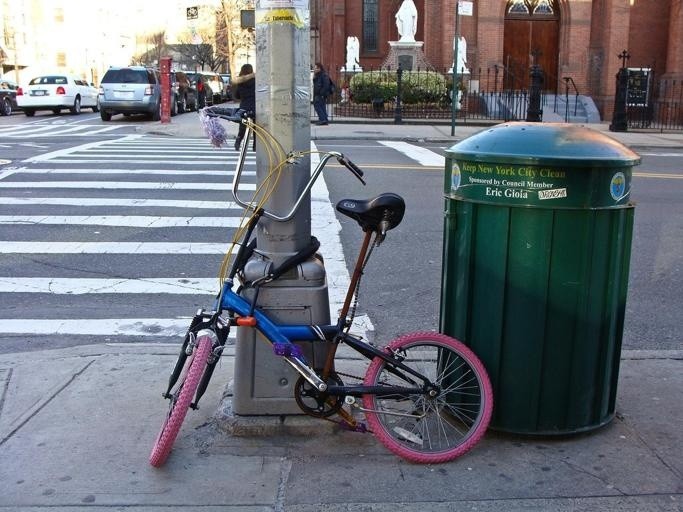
[328,77,335,96]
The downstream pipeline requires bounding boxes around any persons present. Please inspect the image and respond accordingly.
[313,63,330,125]
[234,64,256,152]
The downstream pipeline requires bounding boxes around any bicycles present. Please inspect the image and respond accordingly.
[149,107,494,468]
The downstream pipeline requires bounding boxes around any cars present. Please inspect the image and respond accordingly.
[169,71,232,114]
[15,75,101,117]
[0,81,25,116]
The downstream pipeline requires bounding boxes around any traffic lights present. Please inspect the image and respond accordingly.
[240,10,255,29]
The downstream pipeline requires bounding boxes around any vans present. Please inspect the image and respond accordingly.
[96,65,179,122]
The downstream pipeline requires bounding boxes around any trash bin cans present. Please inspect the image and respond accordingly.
[435,119,643,443]
